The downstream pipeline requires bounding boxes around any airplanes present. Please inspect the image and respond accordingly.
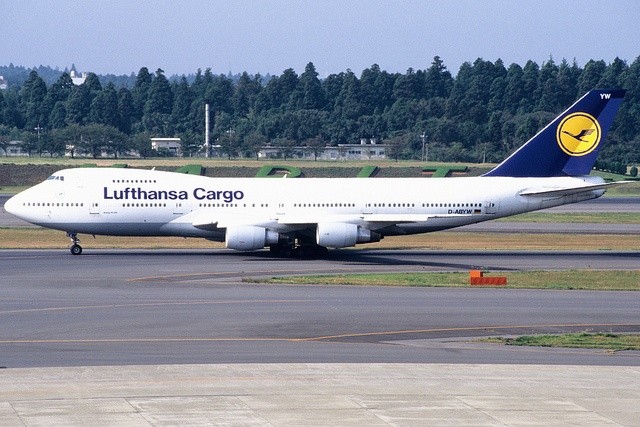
[4,88,635,256]
